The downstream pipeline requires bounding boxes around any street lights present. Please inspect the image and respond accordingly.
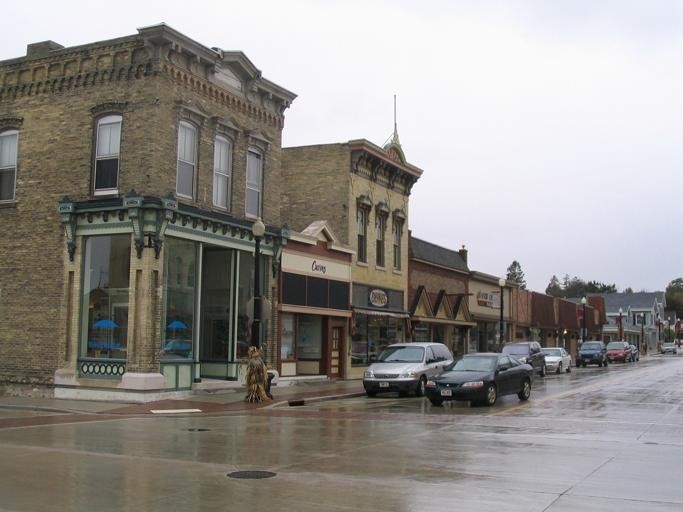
[666,316,670,342]
[639,311,644,355]
[498,277,505,351]
[249,218,267,405]
[677,317,680,348]
[618,306,622,342]
[580,297,586,342]
[657,314,660,351]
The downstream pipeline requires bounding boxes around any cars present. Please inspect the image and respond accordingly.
[362,341,572,408]
[660,343,675,354]
[575,341,638,367]
